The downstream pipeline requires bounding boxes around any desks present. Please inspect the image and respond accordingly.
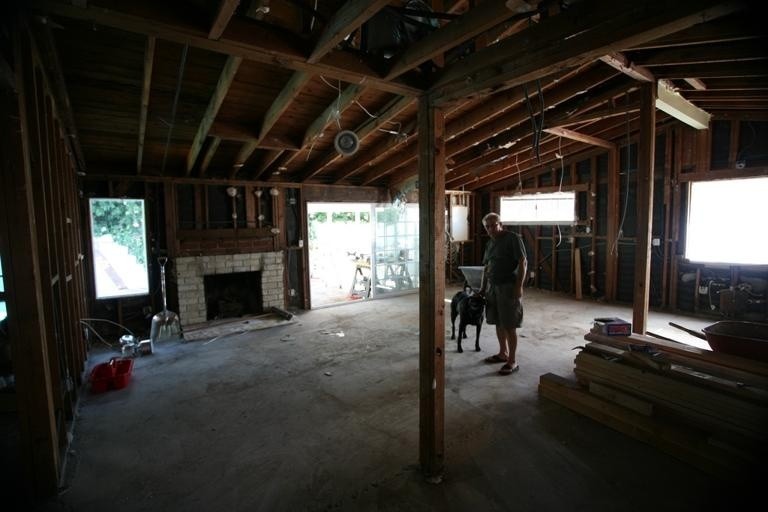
[349,260,414,300]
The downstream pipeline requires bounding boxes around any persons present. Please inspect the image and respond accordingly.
[477,212,527,376]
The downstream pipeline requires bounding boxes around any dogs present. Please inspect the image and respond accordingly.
[450,284,489,353]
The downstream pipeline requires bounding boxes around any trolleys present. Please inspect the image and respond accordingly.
[645,320,767,362]
[458,266,491,297]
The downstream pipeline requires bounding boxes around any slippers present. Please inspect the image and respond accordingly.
[500,364,519,375]
[484,355,507,364]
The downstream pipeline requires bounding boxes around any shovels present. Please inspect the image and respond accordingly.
[150,257,180,343]
